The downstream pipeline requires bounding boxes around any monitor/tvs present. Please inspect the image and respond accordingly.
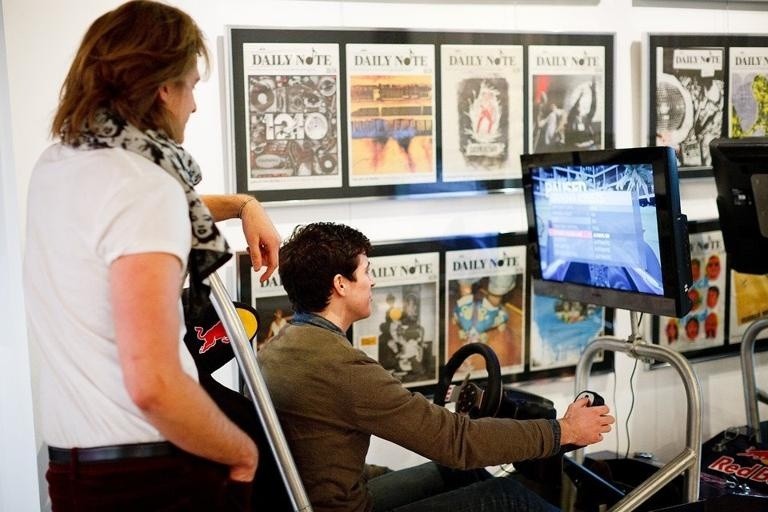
[519,147,694,319]
[709,137,768,276]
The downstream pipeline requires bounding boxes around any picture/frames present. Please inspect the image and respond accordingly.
[642,28,768,179]
[232,232,613,399]
[651,220,768,366]
[226,23,619,209]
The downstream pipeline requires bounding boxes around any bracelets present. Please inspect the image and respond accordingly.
[238,196,256,219]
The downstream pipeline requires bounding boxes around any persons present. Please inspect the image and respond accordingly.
[21,0,281,512]
[456,275,518,339]
[244,223,617,512]
